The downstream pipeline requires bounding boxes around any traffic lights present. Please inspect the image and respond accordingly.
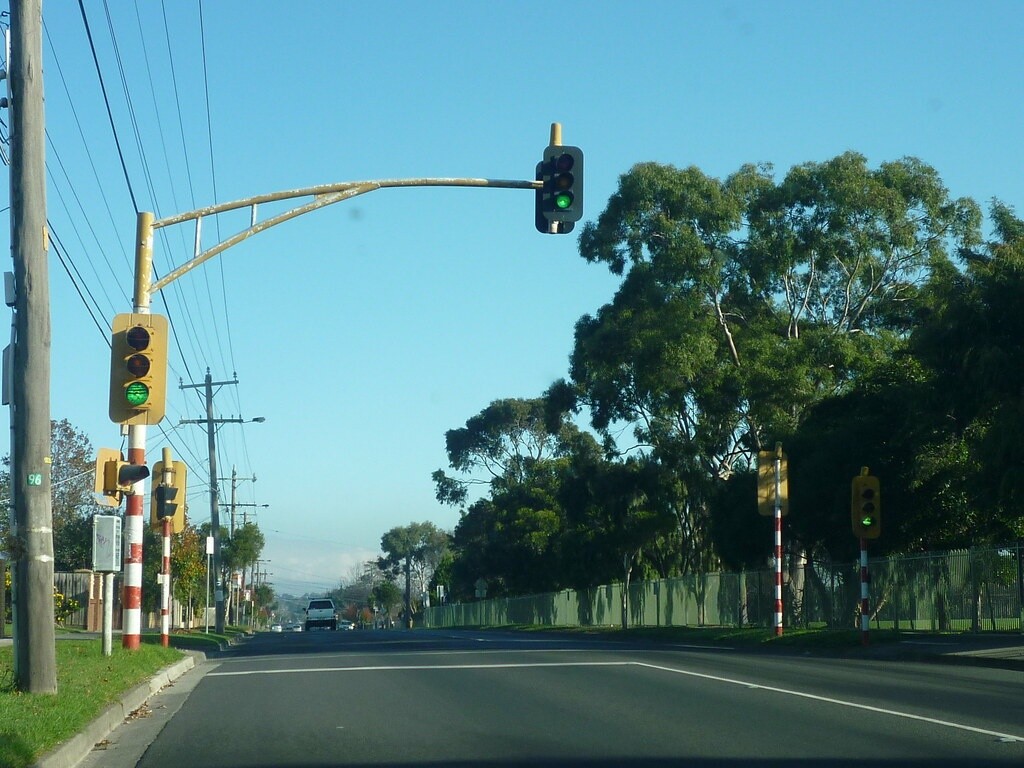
[108,313,168,425]
[116,461,150,491]
[850,475,882,537]
[156,485,178,520]
[541,145,584,222]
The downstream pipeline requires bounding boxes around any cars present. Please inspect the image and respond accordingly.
[293,625,302,632]
[271,624,282,632]
[339,622,355,630]
[286,623,294,630]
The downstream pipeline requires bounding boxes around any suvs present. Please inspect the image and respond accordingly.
[302,597,338,630]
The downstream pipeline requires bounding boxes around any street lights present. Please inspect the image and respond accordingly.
[206,416,266,635]
